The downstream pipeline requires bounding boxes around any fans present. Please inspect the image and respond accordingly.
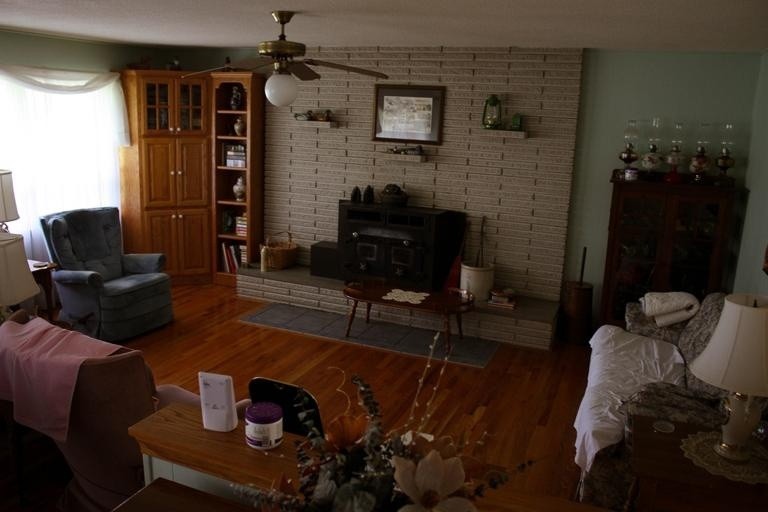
[178,11,389,83]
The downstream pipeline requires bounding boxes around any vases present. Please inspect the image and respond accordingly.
[232,177,246,202]
[459,262,496,302]
[233,117,246,136]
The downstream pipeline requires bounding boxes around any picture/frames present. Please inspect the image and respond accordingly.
[371,83,446,145]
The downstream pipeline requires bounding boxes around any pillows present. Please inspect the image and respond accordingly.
[154,385,200,410]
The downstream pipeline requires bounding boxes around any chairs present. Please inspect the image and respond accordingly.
[39,208,176,340]
[247,377,330,443]
[1,309,252,512]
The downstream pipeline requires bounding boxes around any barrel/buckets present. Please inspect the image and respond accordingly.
[562,281,593,323]
[461,259,498,301]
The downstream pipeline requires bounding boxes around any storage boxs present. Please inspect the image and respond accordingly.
[310,239,338,279]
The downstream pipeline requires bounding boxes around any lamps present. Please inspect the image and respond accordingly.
[263,70,298,106]
[0,170,20,231]
[618,117,735,176]
[1,232,40,319]
[480,94,502,129]
[680,292,767,483]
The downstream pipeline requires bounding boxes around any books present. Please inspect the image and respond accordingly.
[488,295,517,311]
[221,217,247,274]
[215,142,226,166]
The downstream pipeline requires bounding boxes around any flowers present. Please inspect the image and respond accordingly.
[227,327,535,511]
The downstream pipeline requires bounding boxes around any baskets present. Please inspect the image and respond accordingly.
[259,230,298,270]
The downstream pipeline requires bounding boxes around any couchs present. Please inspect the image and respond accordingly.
[569,291,767,502]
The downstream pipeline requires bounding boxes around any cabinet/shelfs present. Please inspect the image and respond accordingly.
[120,136,211,209]
[211,72,267,276]
[336,199,467,302]
[294,111,530,163]
[598,169,751,327]
[122,210,213,274]
[118,68,211,139]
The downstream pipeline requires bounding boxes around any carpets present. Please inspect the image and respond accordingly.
[239,301,496,370]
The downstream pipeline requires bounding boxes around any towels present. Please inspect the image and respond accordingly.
[638,293,701,327]
[1,316,124,441]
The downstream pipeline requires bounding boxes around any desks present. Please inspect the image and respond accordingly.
[618,416,768,510]
[11,256,59,325]
[128,401,329,505]
[110,477,261,512]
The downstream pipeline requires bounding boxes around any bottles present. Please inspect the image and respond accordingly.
[260,244,272,272]
[233,177,246,201]
[234,119,246,136]
